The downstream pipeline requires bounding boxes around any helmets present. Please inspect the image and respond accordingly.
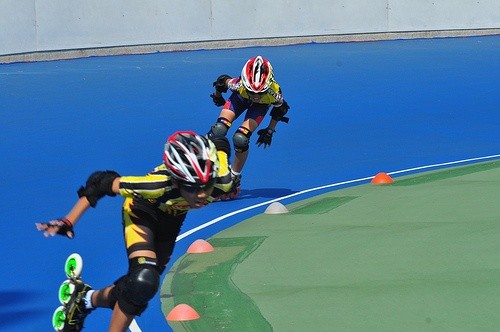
[242,55,274,95]
[164,131,220,184]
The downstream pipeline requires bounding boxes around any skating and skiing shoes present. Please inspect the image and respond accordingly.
[214,171,242,200]
[51,253,93,332]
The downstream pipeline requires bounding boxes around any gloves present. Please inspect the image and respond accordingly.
[256,128,277,149]
[210,93,227,106]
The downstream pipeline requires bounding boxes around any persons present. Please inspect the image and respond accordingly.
[208,55,290,202]
[34,130,231,332]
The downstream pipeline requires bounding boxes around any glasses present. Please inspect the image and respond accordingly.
[178,177,218,193]
[247,90,265,95]
